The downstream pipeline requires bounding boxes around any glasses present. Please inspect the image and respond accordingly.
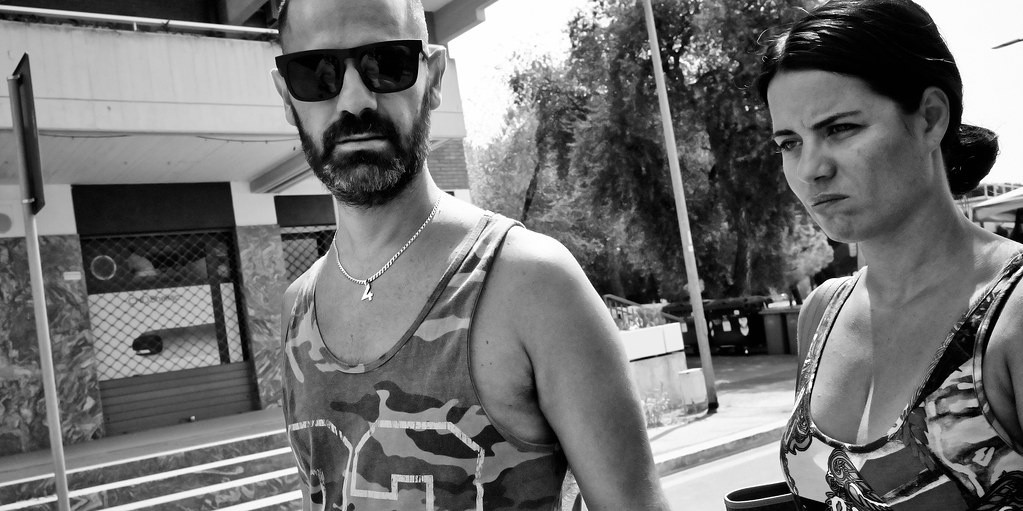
[275,40,431,102]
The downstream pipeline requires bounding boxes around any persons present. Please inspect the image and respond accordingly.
[272,1,668,511]
[756,0,1023,511]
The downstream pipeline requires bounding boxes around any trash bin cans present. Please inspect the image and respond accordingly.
[786,305,801,357]
[698,295,770,355]
[664,300,704,357]
[758,306,788,356]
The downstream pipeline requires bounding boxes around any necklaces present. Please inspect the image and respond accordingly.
[332,185,441,302]
